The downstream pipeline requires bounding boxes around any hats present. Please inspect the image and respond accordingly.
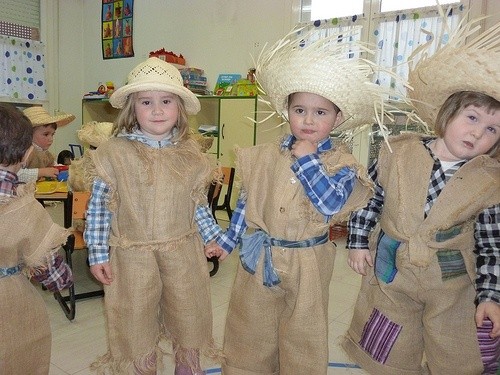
[78,120,113,148]
[109,58,202,115]
[246,26,414,143]
[398,0,499,135]
[24,106,75,129]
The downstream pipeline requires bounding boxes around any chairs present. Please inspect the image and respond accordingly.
[203,167,235,278]
[53,191,105,321]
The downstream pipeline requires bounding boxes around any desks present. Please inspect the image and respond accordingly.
[34,182,73,269]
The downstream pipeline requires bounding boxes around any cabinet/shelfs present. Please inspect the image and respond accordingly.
[81,97,256,211]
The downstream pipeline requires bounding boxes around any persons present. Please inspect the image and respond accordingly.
[346,91,500,375]
[204,92,373,375]
[18,107,75,182]
[83,57,224,375]
[0,103,72,375]
